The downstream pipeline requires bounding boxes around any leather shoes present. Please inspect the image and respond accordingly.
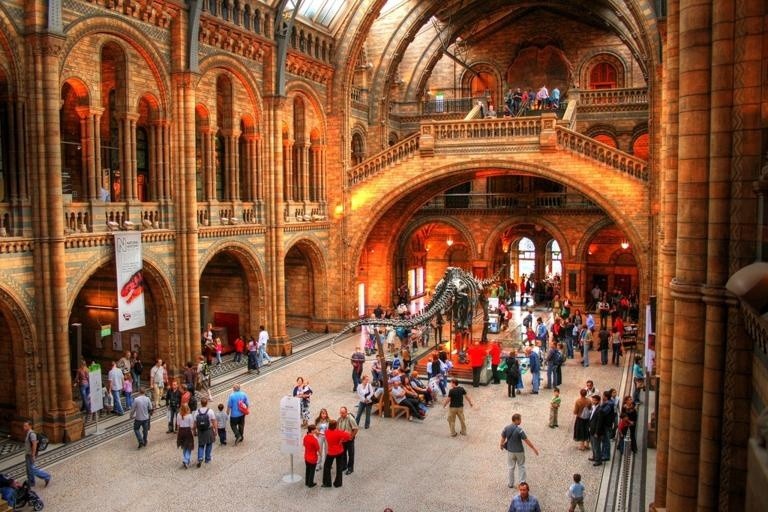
[576,446,609,466]
[530,390,537,394]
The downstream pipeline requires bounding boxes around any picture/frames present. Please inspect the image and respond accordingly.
[94,330,142,355]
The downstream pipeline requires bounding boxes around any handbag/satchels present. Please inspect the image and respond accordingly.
[580,406,591,420]
[365,392,378,404]
[201,336,207,345]
[238,400,249,415]
[188,395,197,411]
[634,377,646,389]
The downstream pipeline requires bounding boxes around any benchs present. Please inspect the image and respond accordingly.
[365,384,424,420]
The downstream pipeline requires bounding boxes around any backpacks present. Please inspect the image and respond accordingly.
[28,432,49,452]
[132,359,143,375]
[199,361,210,376]
[197,408,210,431]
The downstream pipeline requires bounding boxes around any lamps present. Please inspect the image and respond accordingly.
[724,260,768,317]
[335,201,344,213]
[424,233,629,255]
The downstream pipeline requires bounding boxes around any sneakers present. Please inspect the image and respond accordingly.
[166,429,178,434]
[301,423,309,429]
[418,415,425,420]
[111,406,131,416]
[44,475,50,487]
[138,440,146,448]
[635,400,643,405]
[183,458,211,468]
[549,424,558,428]
[451,431,466,436]
[235,434,242,445]
[306,464,352,488]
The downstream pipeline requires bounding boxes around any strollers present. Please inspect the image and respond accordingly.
[0,474,44,511]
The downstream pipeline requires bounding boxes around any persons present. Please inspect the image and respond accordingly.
[483,103,497,137]
[427,351,452,396]
[522,308,535,346]
[233,336,244,362]
[214,338,223,366]
[509,480,542,512]
[384,507,393,512]
[498,412,540,489]
[490,340,501,384]
[520,272,561,308]
[293,377,314,428]
[471,337,483,387]
[130,387,153,448]
[591,284,640,366]
[550,295,594,367]
[151,358,170,409]
[75,350,142,416]
[202,323,214,366]
[1,476,19,512]
[303,406,358,488]
[477,83,562,120]
[499,301,509,328]
[354,373,374,428]
[333,406,360,476]
[166,355,248,469]
[246,325,271,375]
[320,418,354,488]
[549,389,561,428]
[489,276,517,305]
[526,342,566,394]
[302,423,323,489]
[505,351,519,398]
[360,301,429,355]
[22,419,52,488]
[565,473,587,512]
[536,319,547,353]
[370,354,436,420]
[633,356,644,405]
[443,378,472,436]
[502,105,516,135]
[351,347,365,391]
[573,380,638,466]
